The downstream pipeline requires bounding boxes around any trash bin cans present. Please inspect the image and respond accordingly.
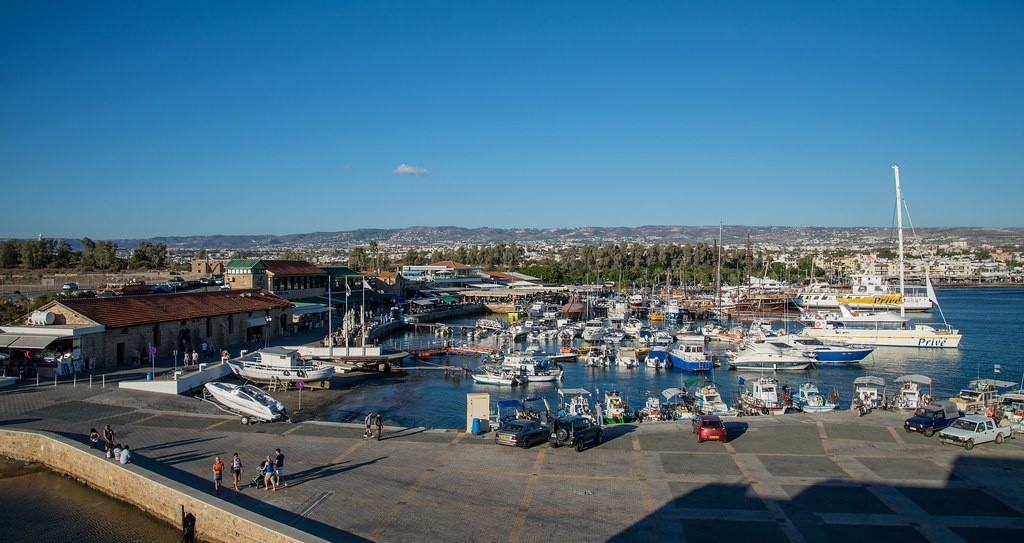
[471,418,481,435]
[147,371,153,380]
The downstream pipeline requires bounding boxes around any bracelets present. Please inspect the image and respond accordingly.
[241,469,243,470]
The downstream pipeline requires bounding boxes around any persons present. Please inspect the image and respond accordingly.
[683,388,686,392]
[289,324,298,335]
[191,350,198,368]
[107,443,114,458]
[231,453,244,491]
[90,428,101,449]
[365,310,374,319]
[212,457,224,491]
[31,361,37,378]
[120,445,136,464]
[114,444,122,461]
[595,400,605,426]
[363,412,374,438]
[991,415,1015,439]
[200,340,214,358]
[260,448,287,491]
[103,425,114,447]
[374,413,383,440]
[184,352,190,366]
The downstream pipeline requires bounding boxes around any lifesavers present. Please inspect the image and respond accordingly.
[904,384,908,389]
[762,407,768,414]
[284,370,289,376]
[297,370,304,377]
[816,322,820,326]
[815,315,820,319]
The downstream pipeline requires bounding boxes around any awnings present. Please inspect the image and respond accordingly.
[246,317,267,328]
[0,335,56,349]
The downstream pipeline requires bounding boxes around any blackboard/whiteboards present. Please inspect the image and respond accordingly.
[61,363,70,374]
[73,360,81,371]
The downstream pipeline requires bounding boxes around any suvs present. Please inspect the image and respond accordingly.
[549,416,602,452]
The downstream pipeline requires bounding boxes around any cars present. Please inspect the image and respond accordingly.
[495,420,549,449]
[63,283,78,291]
[692,416,727,443]
[148,277,231,292]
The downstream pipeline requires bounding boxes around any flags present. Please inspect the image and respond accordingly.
[328,280,373,297]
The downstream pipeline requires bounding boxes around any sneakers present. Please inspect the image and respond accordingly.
[370,435,374,438]
[363,434,367,438]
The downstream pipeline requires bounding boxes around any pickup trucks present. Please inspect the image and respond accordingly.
[904,402,1010,450]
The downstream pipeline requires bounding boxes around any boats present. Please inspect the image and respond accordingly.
[227,347,334,382]
[471,163,1024,434]
[205,382,287,423]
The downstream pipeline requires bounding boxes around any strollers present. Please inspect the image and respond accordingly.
[249,468,264,489]
